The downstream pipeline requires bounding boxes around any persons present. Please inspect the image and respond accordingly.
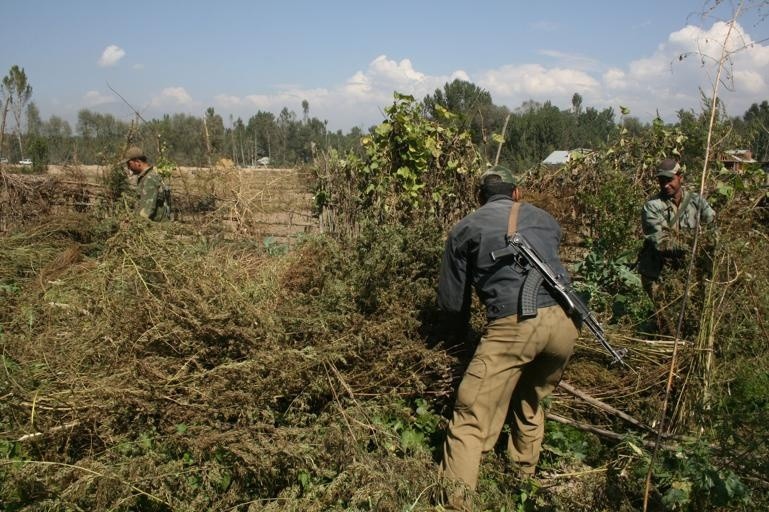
[715,148,769,222]
[256,152,269,169]
[214,153,235,167]
[117,146,170,221]
[437,163,584,511]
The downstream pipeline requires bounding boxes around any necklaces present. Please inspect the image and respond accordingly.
[641,157,718,306]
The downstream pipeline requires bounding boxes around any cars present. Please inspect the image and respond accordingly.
[19,159,32,165]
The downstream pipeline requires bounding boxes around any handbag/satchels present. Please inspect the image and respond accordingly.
[637,241,665,282]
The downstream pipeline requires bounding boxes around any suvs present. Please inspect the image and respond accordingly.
[0,157,9,165]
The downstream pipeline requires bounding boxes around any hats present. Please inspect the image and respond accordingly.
[119,146,145,165]
[655,159,682,179]
[478,164,518,187]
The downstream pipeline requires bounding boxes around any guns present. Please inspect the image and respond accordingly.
[490,232,629,371]
